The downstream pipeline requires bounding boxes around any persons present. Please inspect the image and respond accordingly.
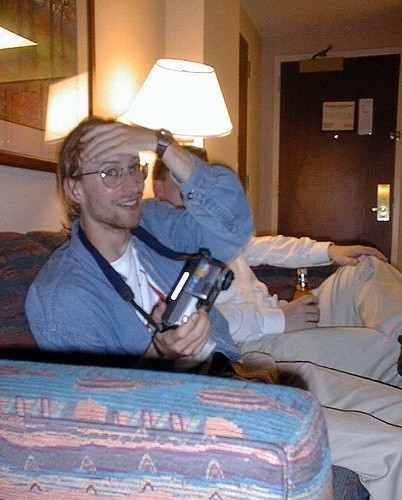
[22,117,402,500]
[151,144,402,388]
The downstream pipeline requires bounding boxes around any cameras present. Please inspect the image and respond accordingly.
[162,249,236,329]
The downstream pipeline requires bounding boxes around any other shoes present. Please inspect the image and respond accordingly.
[396,335,402,377]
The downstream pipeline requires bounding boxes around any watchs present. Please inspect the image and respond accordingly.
[155,129,174,158]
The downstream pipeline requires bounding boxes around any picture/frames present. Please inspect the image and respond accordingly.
[0,0,97,173]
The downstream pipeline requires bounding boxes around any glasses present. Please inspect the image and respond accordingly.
[72,162,149,188]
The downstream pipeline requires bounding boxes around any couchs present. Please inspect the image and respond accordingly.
[0,230,373,500]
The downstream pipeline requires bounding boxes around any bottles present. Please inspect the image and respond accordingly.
[294,269,314,306]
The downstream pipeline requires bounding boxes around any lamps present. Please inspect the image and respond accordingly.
[123,57,234,147]
